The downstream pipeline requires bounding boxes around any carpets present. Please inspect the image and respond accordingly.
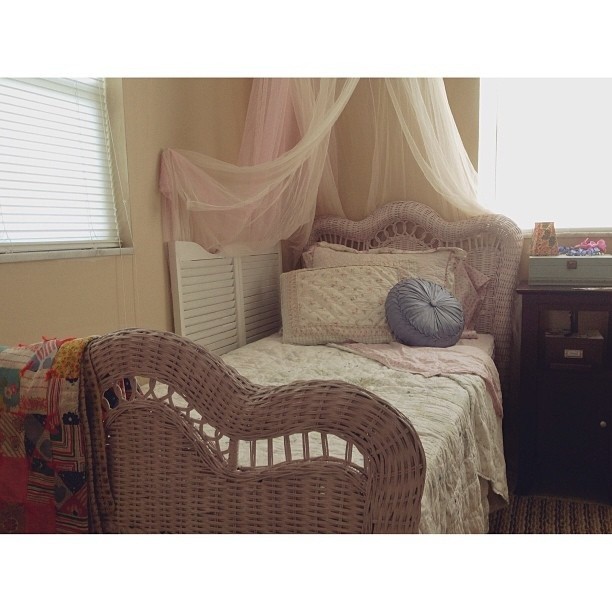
[482,469,612,532]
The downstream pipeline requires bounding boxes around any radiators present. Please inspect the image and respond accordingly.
[170,240,284,358]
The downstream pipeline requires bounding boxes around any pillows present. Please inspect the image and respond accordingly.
[302,238,489,343]
[382,275,465,346]
[277,261,423,345]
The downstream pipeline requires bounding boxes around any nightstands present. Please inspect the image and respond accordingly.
[515,274,611,426]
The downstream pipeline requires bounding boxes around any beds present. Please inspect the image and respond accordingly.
[0,202,524,533]
